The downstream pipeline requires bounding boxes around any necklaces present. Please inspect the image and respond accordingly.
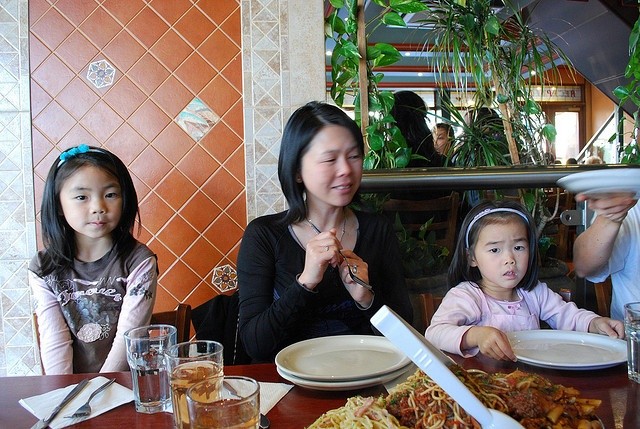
[305,211,347,243]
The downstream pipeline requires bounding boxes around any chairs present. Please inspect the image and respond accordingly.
[33,303,191,376]
[418,288,572,336]
[485,192,573,261]
[594,275,612,319]
[370,192,458,277]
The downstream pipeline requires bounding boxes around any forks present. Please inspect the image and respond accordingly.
[63,377,116,418]
[305,216,373,291]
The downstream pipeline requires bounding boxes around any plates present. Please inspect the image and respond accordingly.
[558,168,640,199]
[275,334,412,381]
[276,363,415,391]
[506,330,631,370]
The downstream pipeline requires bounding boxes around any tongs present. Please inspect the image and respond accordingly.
[368,304,527,428]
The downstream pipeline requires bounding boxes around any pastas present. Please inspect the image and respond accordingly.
[397,365,513,429]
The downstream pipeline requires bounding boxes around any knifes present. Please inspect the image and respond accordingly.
[30,378,90,429]
[223,380,269,429]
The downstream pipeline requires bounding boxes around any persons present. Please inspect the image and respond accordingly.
[450,109,530,167]
[572,192,640,323]
[431,123,453,155]
[425,198,624,363]
[388,91,454,168]
[584,156,603,165]
[567,158,577,164]
[236,101,414,365]
[543,151,555,165]
[28,144,160,375]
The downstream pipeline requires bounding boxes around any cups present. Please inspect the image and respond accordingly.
[164,340,225,429]
[185,376,260,429]
[123,324,178,414]
[623,302,640,384]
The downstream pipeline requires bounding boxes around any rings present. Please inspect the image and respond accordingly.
[325,245,330,251]
[354,265,357,273]
